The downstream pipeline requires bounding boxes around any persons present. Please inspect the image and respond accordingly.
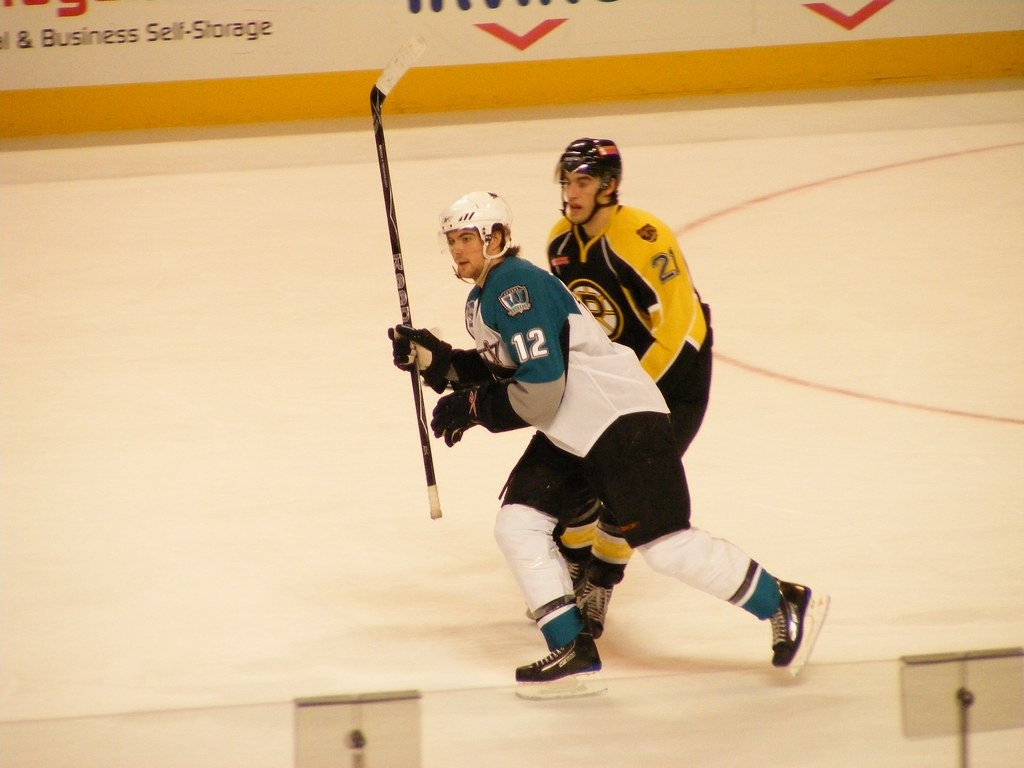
[525,138,713,638]
[387,192,812,685]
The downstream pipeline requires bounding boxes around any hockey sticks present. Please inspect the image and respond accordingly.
[371,36,443,519]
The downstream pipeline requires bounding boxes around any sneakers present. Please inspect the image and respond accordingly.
[768,575,829,679]
[577,576,614,641]
[527,557,586,620]
[514,611,607,699]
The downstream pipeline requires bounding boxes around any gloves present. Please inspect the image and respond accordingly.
[388,325,452,394]
[429,384,490,448]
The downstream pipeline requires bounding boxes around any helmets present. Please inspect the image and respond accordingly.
[559,138,622,207]
[440,191,512,261]
